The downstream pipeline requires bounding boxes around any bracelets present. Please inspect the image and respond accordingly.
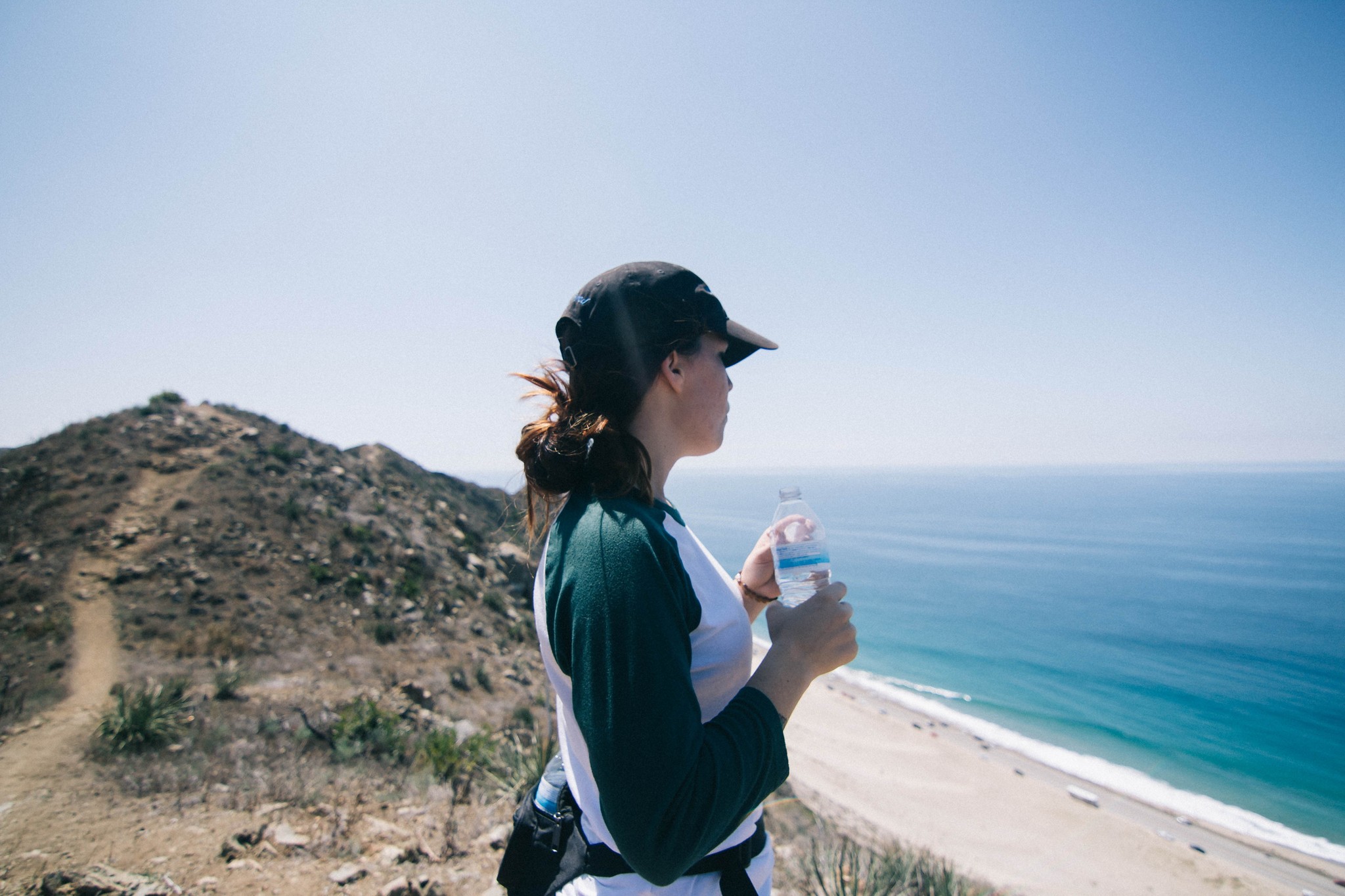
[735,570,778,603]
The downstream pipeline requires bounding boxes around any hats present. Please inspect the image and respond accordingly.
[555,262,779,370]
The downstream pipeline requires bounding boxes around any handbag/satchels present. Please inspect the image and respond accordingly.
[497,776,766,896]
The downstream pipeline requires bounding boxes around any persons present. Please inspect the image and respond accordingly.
[497,262,859,896]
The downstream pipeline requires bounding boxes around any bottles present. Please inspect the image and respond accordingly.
[536,751,566,815]
[770,483,831,608]
